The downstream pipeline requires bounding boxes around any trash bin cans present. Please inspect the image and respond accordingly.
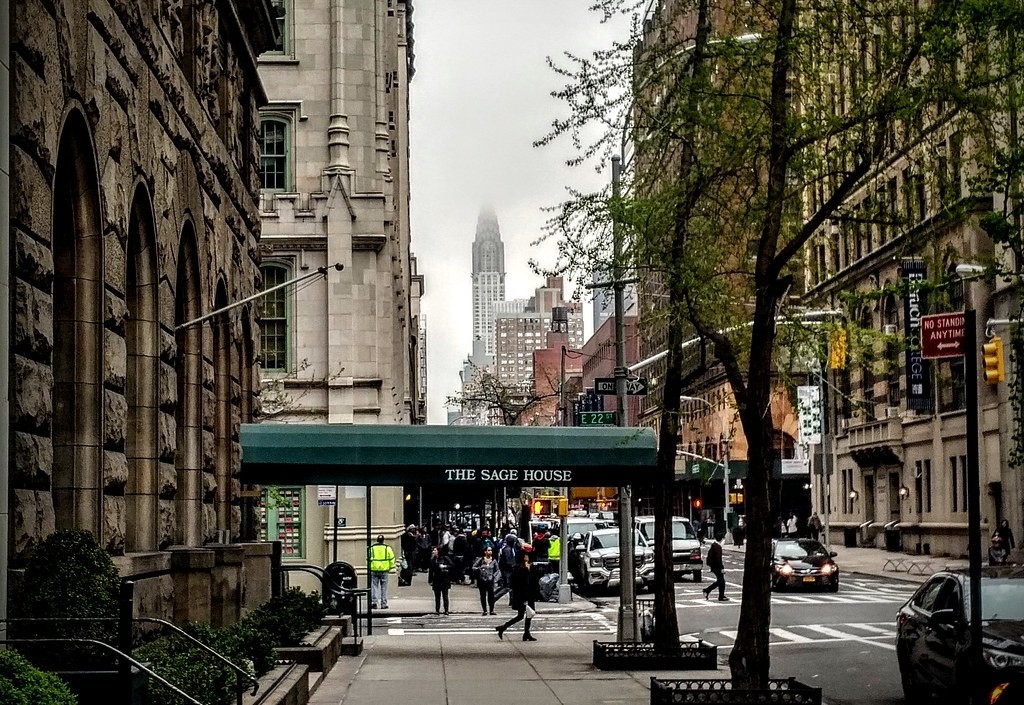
[635,598,655,643]
[320,561,358,623]
[884,529,900,552]
[844,528,857,548]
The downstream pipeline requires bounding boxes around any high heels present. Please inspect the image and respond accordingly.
[495,626,505,640]
[523,633,537,642]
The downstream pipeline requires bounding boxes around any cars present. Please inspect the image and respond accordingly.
[576,531,654,593]
[509,510,619,558]
[449,514,516,531]
[770,538,840,592]
[895,562,1024,705]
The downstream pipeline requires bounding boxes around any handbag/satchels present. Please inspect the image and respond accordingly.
[524,601,535,618]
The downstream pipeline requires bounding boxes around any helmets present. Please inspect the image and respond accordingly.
[992,537,1002,544]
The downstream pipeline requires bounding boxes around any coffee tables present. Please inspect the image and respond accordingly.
[907,560,935,575]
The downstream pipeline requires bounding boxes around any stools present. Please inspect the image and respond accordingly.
[881,557,908,571]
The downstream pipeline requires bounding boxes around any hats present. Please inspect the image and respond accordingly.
[409,524,415,529]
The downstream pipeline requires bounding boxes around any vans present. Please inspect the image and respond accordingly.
[634,516,703,583]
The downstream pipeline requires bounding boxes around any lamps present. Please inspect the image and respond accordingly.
[849,487,859,503]
[899,482,909,500]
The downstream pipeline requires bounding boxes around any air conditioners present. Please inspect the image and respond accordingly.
[882,325,897,334]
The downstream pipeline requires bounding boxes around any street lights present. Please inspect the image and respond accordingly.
[956,264,988,705]
[680,396,734,544]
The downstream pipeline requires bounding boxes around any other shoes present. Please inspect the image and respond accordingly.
[381,606,388,609]
[719,596,730,602]
[703,588,708,601]
[445,610,449,615]
[483,611,487,616]
[436,611,439,615]
[371,603,377,609]
[490,611,497,616]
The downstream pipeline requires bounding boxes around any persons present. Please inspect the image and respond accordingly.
[365,535,396,609]
[472,545,500,616]
[702,535,730,603]
[428,547,454,616]
[494,550,546,641]
[991,519,1015,556]
[808,512,823,541]
[786,514,798,538]
[704,515,714,539]
[400,522,560,603]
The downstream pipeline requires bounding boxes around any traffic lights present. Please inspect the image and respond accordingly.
[729,493,736,504]
[533,499,552,515]
[981,337,1004,384]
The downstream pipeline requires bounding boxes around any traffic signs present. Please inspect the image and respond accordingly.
[578,411,617,425]
[595,378,648,396]
[920,312,964,359]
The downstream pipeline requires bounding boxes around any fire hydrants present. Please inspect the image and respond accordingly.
[694,499,702,508]
